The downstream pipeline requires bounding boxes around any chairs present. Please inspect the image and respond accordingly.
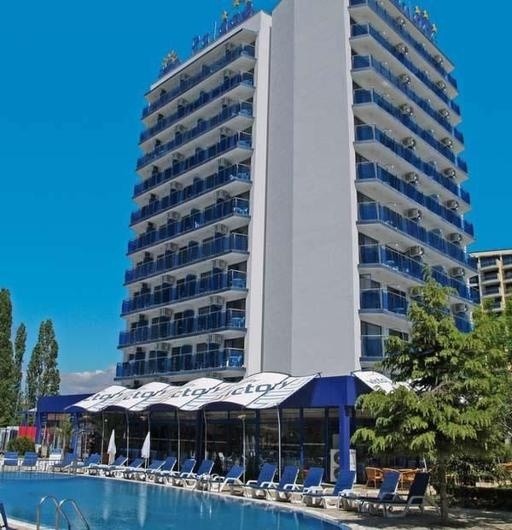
[50,452,166,482]
[303,469,357,509]
[172,459,216,488]
[228,463,278,496]
[20,452,40,475]
[336,469,402,520]
[1,451,21,475]
[357,469,431,519]
[155,458,197,487]
[201,465,248,493]
[278,467,326,504]
[250,466,300,500]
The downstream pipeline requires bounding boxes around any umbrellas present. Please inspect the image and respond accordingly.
[140,431,151,469]
[106,428,116,464]
